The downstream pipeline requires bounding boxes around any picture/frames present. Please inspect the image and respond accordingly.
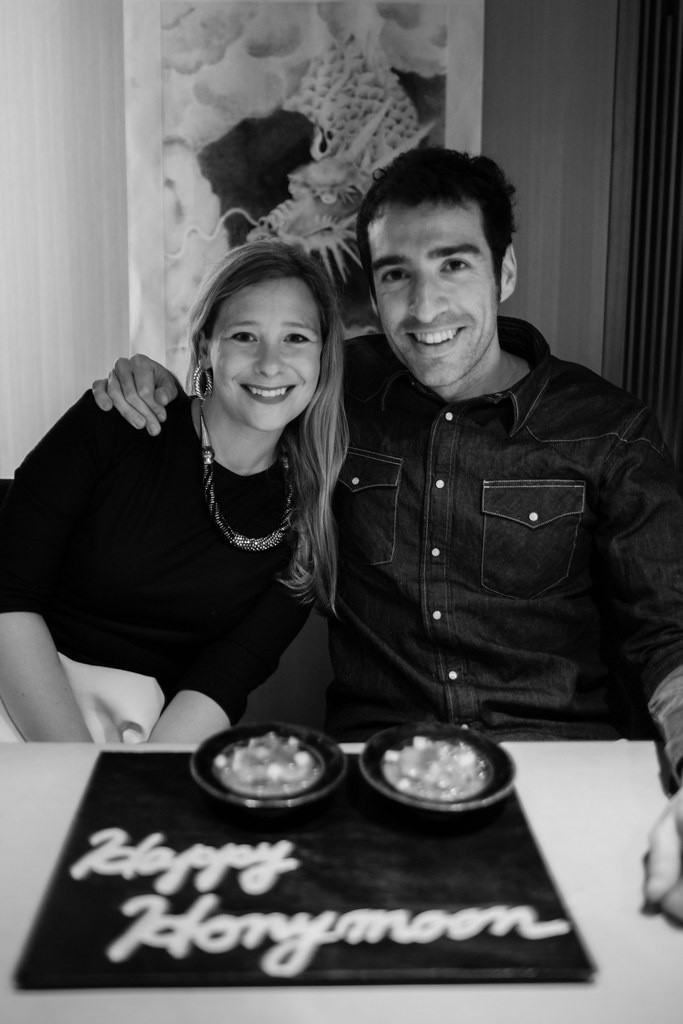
[123,0,483,391]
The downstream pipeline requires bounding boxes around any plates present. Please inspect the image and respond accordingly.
[190,721,346,809]
[357,724,517,812]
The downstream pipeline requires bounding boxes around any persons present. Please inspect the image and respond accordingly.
[0,239,351,746]
[92,145,683,925]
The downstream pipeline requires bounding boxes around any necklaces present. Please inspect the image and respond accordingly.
[200,401,294,550]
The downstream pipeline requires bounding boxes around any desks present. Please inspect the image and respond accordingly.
[1,737,683,1024]
